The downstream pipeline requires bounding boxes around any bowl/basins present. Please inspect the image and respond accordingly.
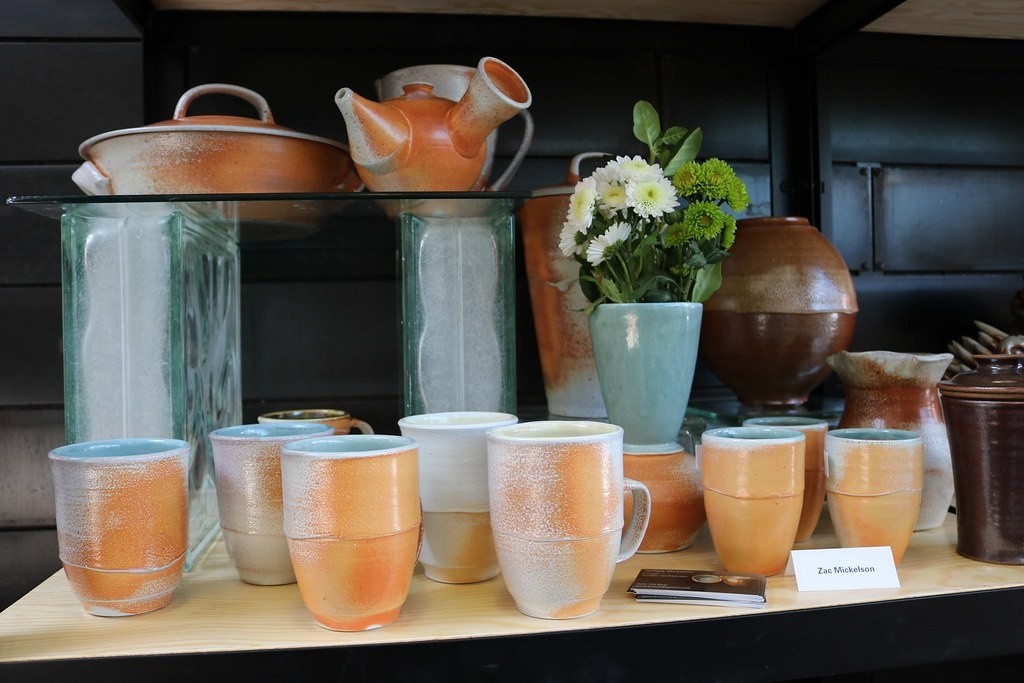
[71,83,367,253]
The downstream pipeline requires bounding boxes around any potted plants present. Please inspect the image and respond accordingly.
[584,298,708,456]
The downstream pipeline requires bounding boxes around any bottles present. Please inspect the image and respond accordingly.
[696,218,860,407]
[936,354,1023,566]
[519,151,611,420]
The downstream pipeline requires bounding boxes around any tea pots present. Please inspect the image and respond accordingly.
[335,54,532,218]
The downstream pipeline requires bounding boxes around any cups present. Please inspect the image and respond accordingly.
[824,429,924,568]
[398,411,519,583]
[281,434,422,633]
[208,424,335,585]
[486,419,653,620]
[376,64,534,216]
[745,417,830,542]
[258,410,374,436]
[47,436,191,616]
[824,350,955,531]
[701,427,805,578]
